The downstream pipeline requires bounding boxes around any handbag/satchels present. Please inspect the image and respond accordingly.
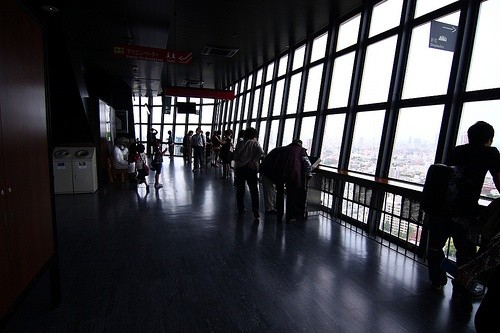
[142,164,149,173]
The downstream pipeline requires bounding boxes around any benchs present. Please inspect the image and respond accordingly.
[107,157,126,182]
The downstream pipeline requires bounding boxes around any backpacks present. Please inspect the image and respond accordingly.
[419,163,470,218]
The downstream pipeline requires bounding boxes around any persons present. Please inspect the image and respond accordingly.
[151,142,168,189]
[427,122,500,333]
[183,128,234,172]
[259,146,290,213]
[285,139,312,222]
[147,128,158,157]
[167,131,174,158]
[234,126,262,218]
[111,135,150,192]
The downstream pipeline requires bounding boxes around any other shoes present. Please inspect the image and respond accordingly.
[155,183,162,187]
[288,217,295,222]
[453,287,483,299]
[433,281,445,290]
[253,209,260,218]
[239,209,246,214]
[265,209,278,214]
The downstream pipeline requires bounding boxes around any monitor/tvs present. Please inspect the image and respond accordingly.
[177,102,196,114]
